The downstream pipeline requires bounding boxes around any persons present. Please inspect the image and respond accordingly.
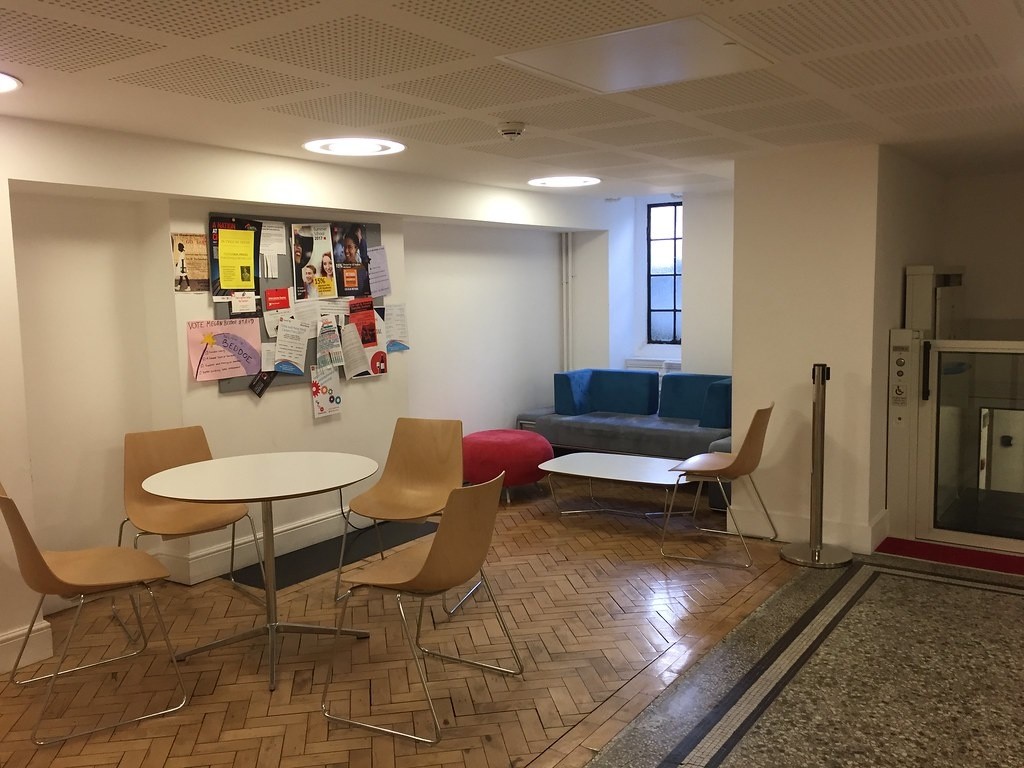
[293,228,313,299]
[241,267,249,281]
[344,223,363,264]
[320,252,333,277]
[305,265,317,292]
[175,243,192,292]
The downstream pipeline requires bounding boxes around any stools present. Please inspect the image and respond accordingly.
[462,429,555,504]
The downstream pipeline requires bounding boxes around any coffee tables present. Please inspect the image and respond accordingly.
[538,452,703,532]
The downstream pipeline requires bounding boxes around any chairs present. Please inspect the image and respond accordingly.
[321,470,527,746]
[1,483,188,746]
[118,425,269,642]
[660,401,779,569]
[333,418,464,603]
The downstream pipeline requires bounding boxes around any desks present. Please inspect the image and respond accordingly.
[142,450,380,693]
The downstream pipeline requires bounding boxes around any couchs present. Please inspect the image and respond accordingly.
[515,366,734,512]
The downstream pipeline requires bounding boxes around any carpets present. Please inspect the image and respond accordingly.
[876,535,1023,576]
